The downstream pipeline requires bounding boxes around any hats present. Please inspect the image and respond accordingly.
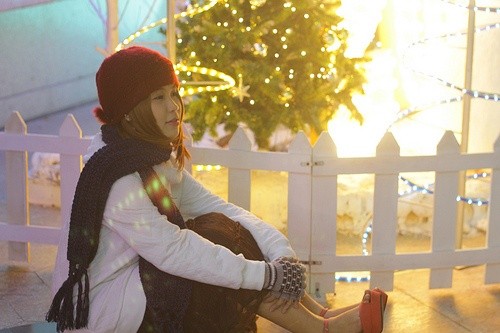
[95,46,180,125]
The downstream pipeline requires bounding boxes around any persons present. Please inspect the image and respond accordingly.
[44,46,389,333]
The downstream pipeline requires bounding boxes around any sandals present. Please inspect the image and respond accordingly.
[321,289,383,333]
[320,287,388,318]
